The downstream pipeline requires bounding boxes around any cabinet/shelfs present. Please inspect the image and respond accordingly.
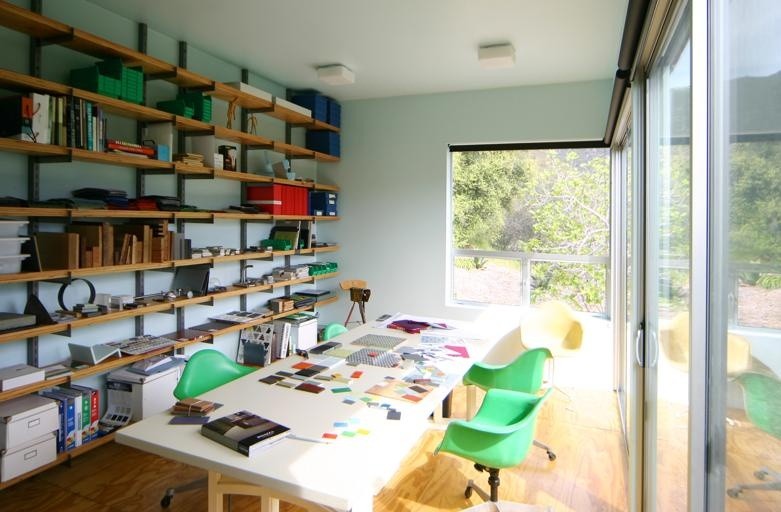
[0,1,340,487]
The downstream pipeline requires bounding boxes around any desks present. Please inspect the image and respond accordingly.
[114,312,507,512]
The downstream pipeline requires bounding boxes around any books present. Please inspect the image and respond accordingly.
[0,91,156,159]
[271,264,309,281]
[38,383,99,454]
[200,409,292,458]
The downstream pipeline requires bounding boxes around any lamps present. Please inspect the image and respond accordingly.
[317,66,355,87]
[477,43,517,71]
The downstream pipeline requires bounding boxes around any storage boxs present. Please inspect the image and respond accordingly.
[287,87,342,155]
[0,394,61,484]
[108,364,180,423]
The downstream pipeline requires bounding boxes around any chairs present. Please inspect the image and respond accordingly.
[320,323,348,344]
[160,348,262,509]
[434,347,558,512]
[661,311,781,498]
